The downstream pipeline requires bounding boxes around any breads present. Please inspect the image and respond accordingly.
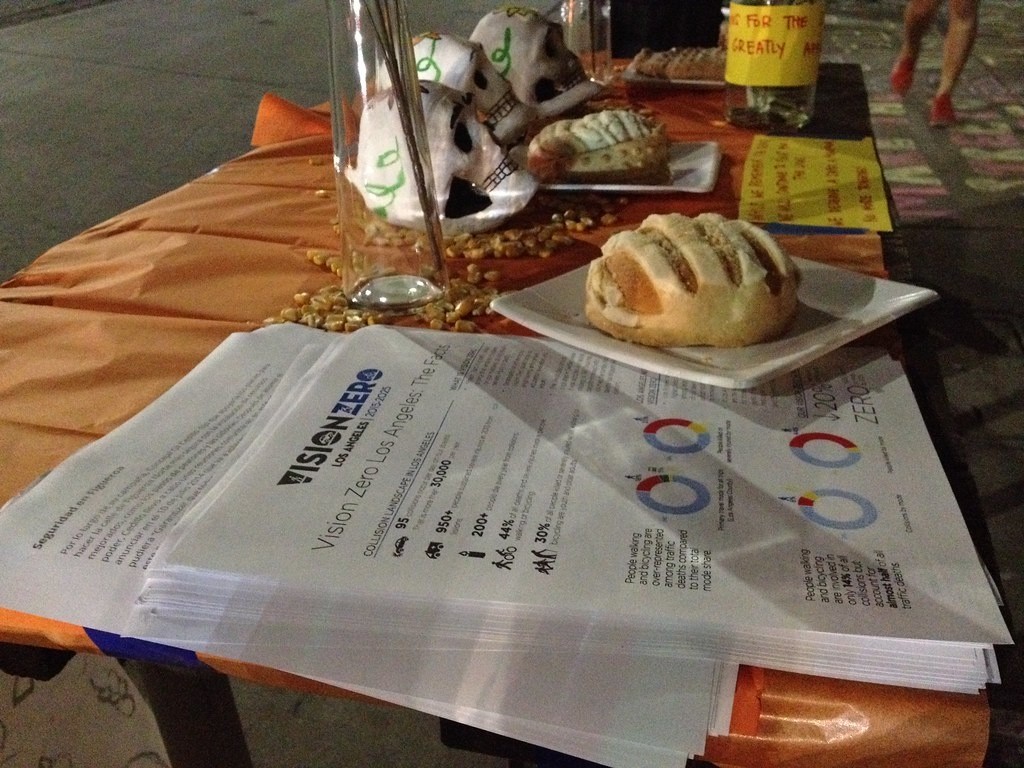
[587,212,805,348]
[527,109,679,184]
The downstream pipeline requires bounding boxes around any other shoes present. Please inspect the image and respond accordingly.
[929,94,956,127]
[890,57,916,98]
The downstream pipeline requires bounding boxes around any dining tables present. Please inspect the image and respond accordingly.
[2,30,996,767]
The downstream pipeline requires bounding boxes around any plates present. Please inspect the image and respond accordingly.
[508,143,723,194]
[620,59,725,89]
[488,257,942,389]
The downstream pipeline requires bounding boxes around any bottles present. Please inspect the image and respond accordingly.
[326,0,453,314]
[724,1,826,132]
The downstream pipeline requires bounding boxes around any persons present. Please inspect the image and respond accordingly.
[891,0,980,127]
